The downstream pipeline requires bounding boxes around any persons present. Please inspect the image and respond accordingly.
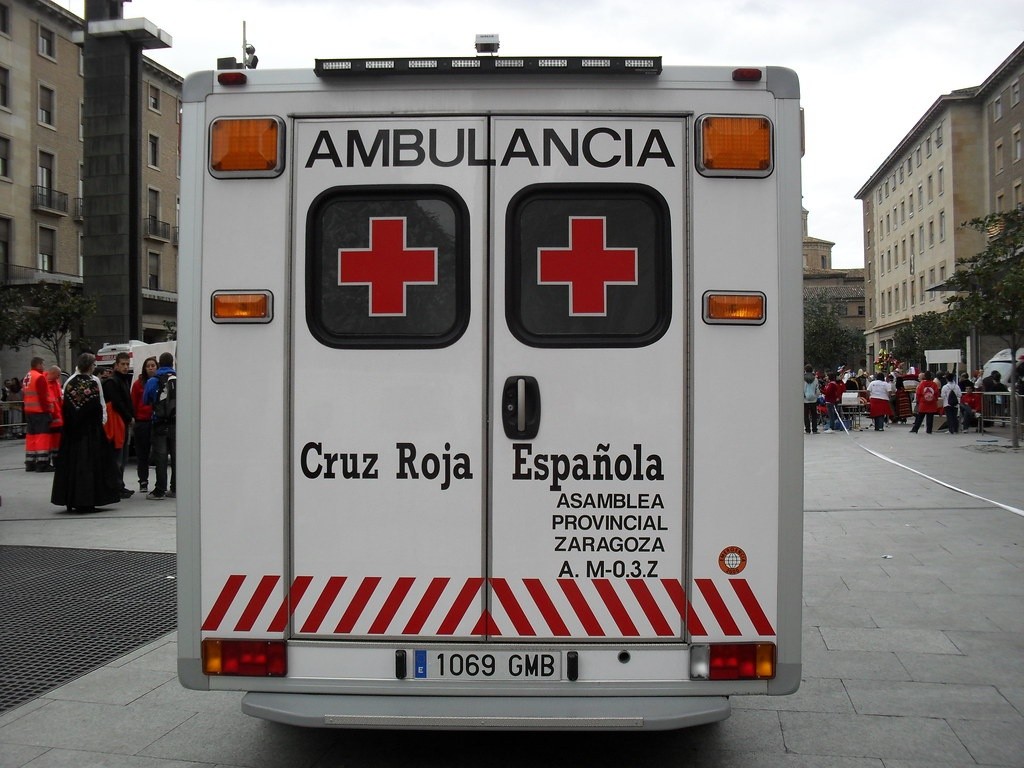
[59,361,119,425]
[804,360,1008,435]
[130,356,160,493]
[50,352,134,513]
[102,352,135,498]
[142,351,176,501]
[22,356,60,474]
[0,377,24,441]
[42,365,63,471]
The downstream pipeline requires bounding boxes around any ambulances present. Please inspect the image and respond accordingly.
[172,32,808,734]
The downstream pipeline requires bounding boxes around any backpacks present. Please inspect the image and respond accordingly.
[151,372,177,419]
[805,383,816,401]
[923,383,934,402]
[946,384,959,406]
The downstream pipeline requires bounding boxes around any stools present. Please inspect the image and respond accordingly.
[846,407,858,428]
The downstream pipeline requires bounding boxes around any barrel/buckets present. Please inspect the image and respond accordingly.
[840,420,850,431]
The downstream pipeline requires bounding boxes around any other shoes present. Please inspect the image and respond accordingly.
[962,429,969,433]
[879,429,884,431]
[139,481,148,492]
[146,488,165,500]
[67,504,96,512]
[975,413,981,418]
[24,462,37,471]
[909,430,917,433]
[813,431,820,434]
[166,490,176,498]
[37,462,55,472]
[945,431,952,434]
[824,428,835,433]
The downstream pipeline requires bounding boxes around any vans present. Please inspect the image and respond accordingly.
[969,348,1024,390]
[95,339,152,370]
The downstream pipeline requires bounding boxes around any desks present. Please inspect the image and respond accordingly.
[837,402,870,429]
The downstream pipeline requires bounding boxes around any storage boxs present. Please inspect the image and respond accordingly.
[834,419,852,430]
[841,392,861,405]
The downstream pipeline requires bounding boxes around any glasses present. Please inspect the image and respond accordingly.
[92,362,98,367]
